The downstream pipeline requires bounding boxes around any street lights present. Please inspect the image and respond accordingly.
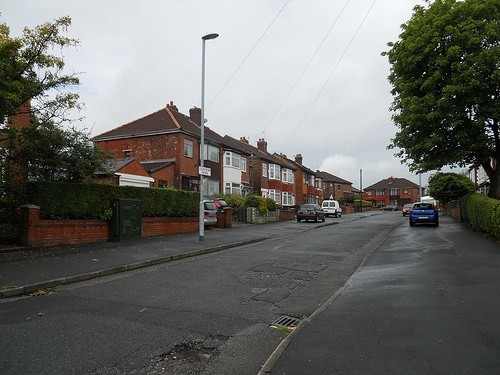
[198,33,220,242]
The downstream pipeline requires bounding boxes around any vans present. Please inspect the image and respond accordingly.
[320,200,342,218]
[421,196,436,205]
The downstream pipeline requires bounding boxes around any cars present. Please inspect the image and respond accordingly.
[203,200,218,228]
[409,202,441,227]
[296,204,326,223]
[211,198,228,212]
[379,204,399,212]
[402,204,413,216]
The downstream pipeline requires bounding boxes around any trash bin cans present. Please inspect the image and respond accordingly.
[110,197,143,241]
[356,204,361,211]
